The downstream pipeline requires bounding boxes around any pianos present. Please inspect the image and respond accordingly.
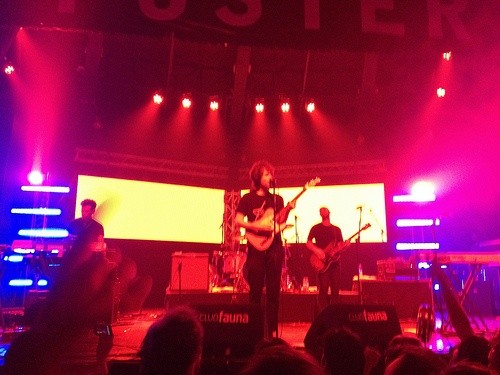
[11,239,107,310]
[410,249,500,335]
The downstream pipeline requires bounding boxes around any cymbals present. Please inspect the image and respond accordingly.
[282,224,294,232]
[232,236,247,245]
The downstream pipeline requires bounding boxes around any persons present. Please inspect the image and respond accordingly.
[67,198,104,245]
[307,208,350,313]
[0,239,500,375]
[236,161,295,334]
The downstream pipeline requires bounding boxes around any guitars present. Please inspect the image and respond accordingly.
[310,222,371,273]
[245,176,320,251]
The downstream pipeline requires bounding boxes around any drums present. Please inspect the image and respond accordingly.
[223,250,247,278]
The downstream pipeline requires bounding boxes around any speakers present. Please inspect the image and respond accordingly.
[345,305,402,346]
[170,252,208,294]
[192,302,260,355]
